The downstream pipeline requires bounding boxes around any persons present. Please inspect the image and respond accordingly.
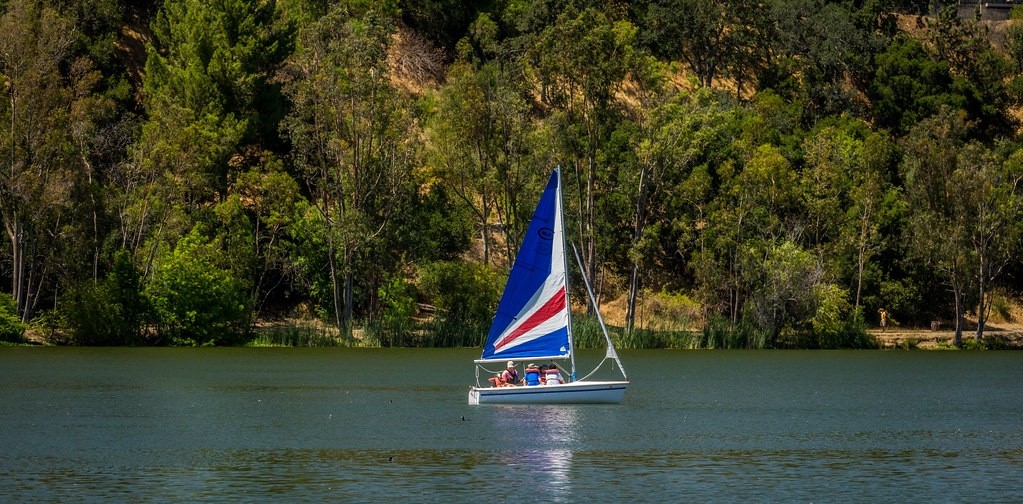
[545,364,565,384]
[539,365,548,382]
[523,363,545,386]
[502,361,524,387]
[878,308,887,333]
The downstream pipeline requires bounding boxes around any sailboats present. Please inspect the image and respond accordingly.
[468,163,630,405]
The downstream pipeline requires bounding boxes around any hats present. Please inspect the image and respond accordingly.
[527,363,538,368]
[507,361,514,368]
